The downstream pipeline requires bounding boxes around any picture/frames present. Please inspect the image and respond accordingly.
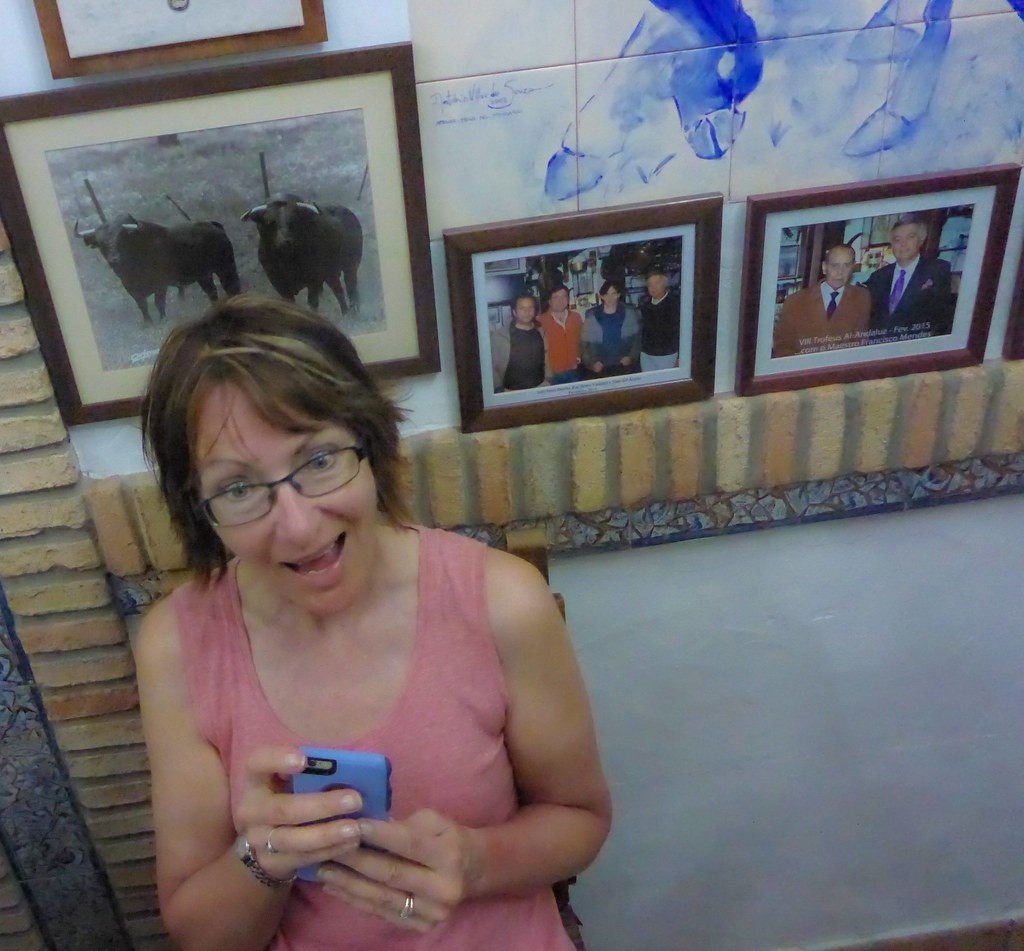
[442,193,724,434]
[0,41,442,426]
[734,162,1022,397]
[31,0,330,81]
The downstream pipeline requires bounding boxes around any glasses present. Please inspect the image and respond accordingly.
[188,438,368,528]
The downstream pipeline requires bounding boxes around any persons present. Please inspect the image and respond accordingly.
[135,293,612,951]
[772,218,951,358]
[490,269,681,392]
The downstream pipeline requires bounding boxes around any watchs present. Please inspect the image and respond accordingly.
[236,833,297,888]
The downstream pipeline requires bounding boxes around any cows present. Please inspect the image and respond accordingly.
[243,191,364,315]
[72,210,242,322]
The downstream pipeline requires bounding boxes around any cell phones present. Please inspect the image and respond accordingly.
[291,746,393,882]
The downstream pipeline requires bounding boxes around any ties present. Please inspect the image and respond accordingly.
[827,292,839,312]
[889,270,905,313]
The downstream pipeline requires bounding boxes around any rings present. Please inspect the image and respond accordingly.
[266,830,278,853]
[399,896,413,920]
